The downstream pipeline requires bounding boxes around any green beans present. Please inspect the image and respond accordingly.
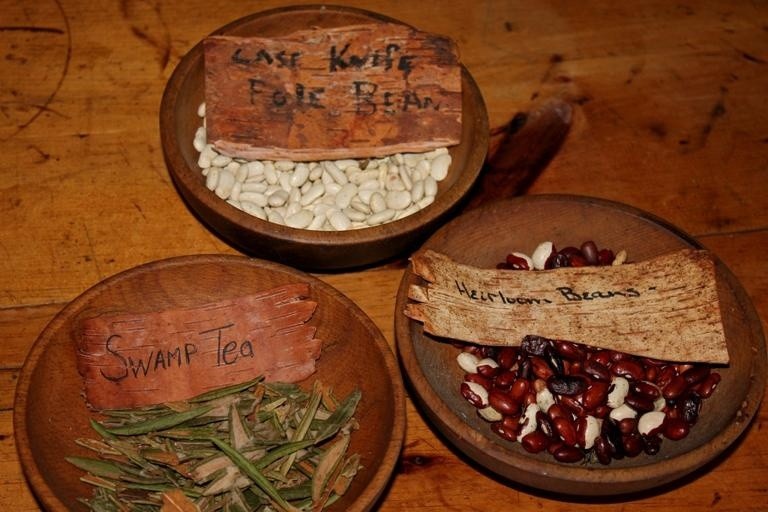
[451,238,721,466]
[192,102,453,231]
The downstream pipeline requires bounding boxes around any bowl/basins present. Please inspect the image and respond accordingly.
[159,6,490,269]
[391,193,768,512]
[16,253,406,511]
